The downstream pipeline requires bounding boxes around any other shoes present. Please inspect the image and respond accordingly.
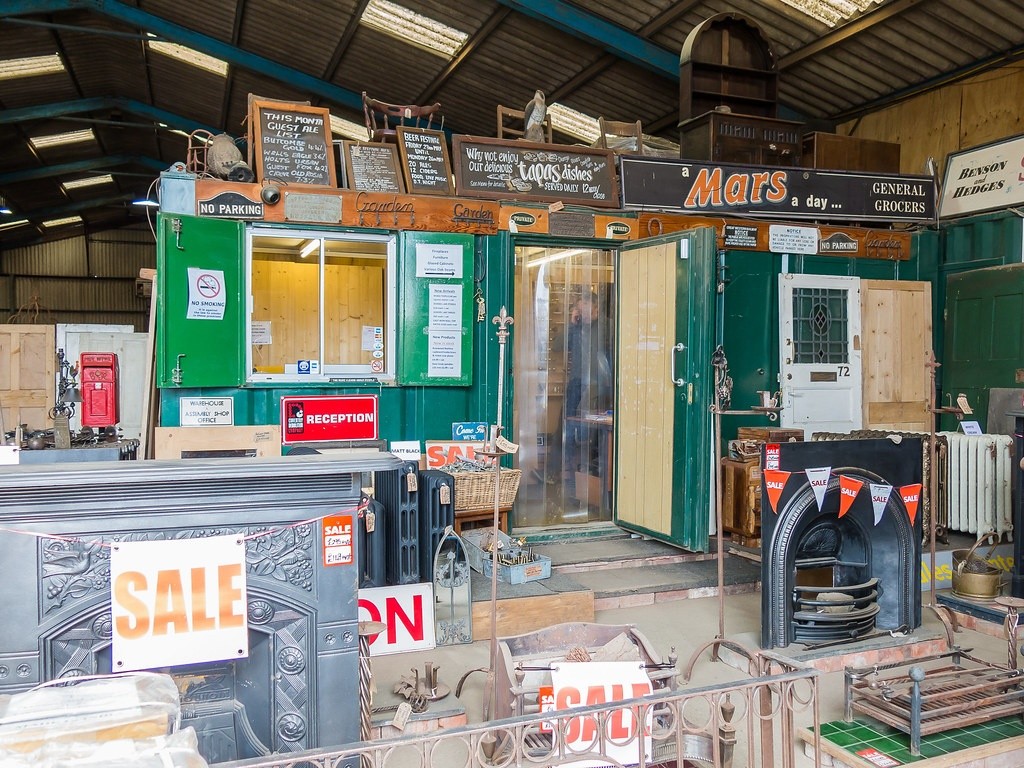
[531,468,555,485]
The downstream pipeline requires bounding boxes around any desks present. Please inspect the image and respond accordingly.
[801,131,902,229]
[564,410,614,520]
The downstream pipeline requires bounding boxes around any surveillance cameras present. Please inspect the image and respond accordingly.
[260,185,281,205]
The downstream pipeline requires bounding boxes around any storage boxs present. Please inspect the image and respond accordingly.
[727,438,767,463]
[461,526,522,575]
[492,622,684,758]
[737,426,805,445]
[480,549,553,586]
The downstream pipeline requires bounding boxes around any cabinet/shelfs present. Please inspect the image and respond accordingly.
[525,278,598,483]
[721,456,763,539]
[676,8,807,169]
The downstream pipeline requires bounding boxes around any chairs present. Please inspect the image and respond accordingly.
[361,89,442,145]
[598,115,644,176]
[497,104,553,144]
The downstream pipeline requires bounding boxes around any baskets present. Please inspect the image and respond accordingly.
[445,465,522,511]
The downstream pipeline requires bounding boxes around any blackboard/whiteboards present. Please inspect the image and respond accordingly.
[252,99,338,189]
[451,133,621,208]
[342,140,406,196]
[396,125,456,197]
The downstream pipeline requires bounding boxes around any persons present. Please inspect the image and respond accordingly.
[530,288,613,487]
